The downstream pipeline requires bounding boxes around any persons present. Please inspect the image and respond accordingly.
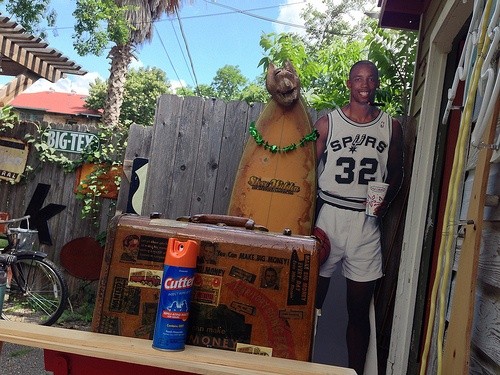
[311,60,404,375]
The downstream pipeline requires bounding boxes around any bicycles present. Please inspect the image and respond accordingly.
[0,214,69,326]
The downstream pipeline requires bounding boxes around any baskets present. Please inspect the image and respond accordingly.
[8,218,39,252]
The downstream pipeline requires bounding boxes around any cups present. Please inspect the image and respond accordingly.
[365,181,390,216]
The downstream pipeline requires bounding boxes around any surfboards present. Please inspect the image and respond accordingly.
[226,94,317,237]
